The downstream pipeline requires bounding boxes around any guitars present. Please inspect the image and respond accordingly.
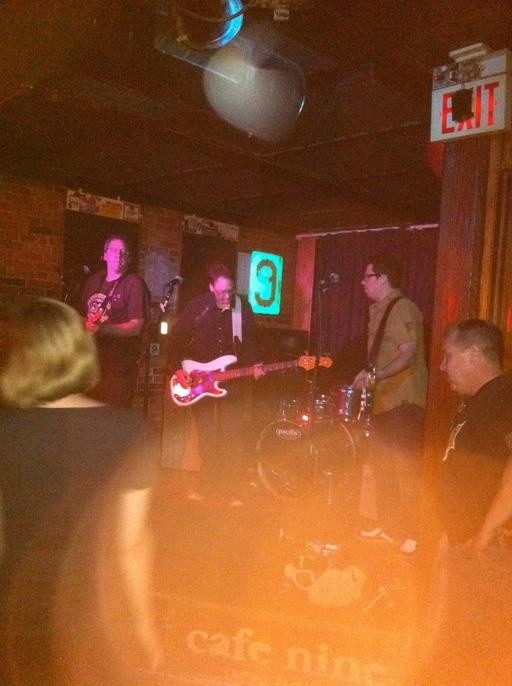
[169,355,334,406]
[347,361,375,421]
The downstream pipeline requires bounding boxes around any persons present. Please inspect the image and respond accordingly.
[350,256,427,554]
[76,232,151,411]
[171,264,268,476]
[1,295,163,686]
[417,319,512,686]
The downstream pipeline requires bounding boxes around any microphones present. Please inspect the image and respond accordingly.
[64,265,90,274]
[163,275,183,289]
[320,272,340,285]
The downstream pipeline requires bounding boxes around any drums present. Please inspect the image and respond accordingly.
[330,384,373,426]
[256,418,358,505]
